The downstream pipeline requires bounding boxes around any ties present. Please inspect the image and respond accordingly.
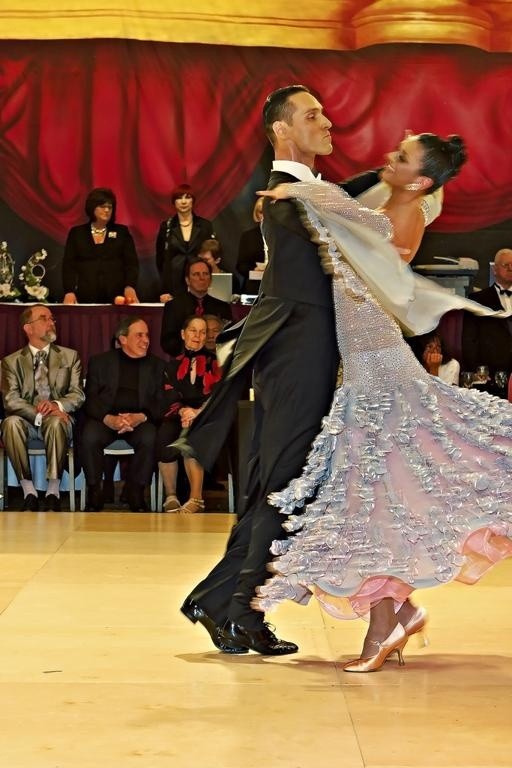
[35,351,50,401]
[195,298,205,316]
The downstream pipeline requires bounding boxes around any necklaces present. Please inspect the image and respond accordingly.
[179,218,193,227]
[91,224,106,236]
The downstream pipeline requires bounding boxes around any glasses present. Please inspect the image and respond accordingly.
[26,317,57,324]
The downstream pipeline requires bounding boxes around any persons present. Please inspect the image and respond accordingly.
[159,237,241,304]
[159,256,233,357]
[155,184,213,303]
[236,196,268,296]
[412,330,459,388]
[178,84,416,659]
[203,314,225,352]
[461,246,512,401]
[75,316,178,511]
[1,304,85,511]
[249,129,460,674]
[60,186,141,305]
[155,315,218,515]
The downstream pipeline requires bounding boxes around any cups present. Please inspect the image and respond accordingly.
[460,364,508,389]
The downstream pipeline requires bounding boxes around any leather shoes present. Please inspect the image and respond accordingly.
[219,611,299,656]
[180,595,249,654]
[22,493,60,512]
[85,480,146,512]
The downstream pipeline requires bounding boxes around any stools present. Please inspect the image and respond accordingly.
[158,443,237,514]
[2,427,78,514]
[78,431,162,512]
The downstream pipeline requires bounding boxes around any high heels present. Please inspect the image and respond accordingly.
[342,606,428,674]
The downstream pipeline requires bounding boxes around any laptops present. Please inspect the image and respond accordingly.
[188,273,238,304]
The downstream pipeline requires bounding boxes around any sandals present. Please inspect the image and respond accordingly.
[163,495,206,514]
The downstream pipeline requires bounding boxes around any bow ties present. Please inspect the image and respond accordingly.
[499,289,512,296]
